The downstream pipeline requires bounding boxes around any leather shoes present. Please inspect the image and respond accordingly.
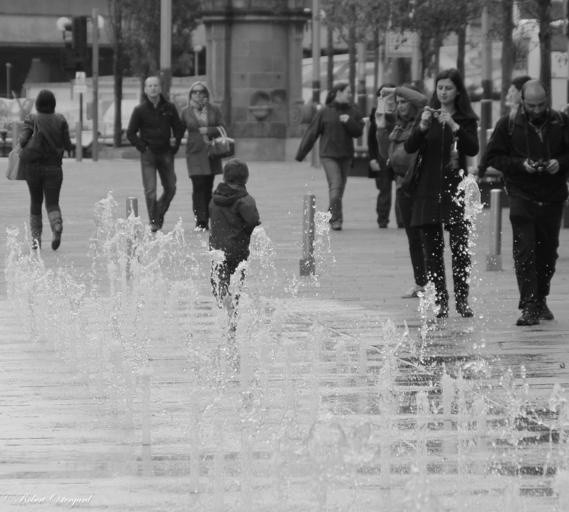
[517,310,540,326]
[540,304,553,319]
[436,303,450,318]
[457,300,473,317]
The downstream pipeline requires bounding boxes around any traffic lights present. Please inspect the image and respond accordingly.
[64,15,87,57]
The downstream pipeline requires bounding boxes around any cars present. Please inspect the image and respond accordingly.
[302,42,503,104]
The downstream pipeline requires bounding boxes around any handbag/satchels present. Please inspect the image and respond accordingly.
[207,126,235,160]
[19,116,43,164]
[402,148,423,198]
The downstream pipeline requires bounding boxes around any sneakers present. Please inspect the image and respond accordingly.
[333,221,342,230]
[403,285,424,297]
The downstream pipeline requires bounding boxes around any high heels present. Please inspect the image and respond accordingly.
[52,223,62,250]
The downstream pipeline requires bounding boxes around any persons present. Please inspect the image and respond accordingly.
[124,76,184,231]
[476,76,529,185]
[386,86,429,301]
[294,84,364,232]
[176,80,225,233]
[501,80,567,326]
[367,84,410,228]
[11,89,73,251]
[400,69,481,319]
[207,156,260,300]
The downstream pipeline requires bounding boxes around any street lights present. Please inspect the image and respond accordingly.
[57,8,105,161]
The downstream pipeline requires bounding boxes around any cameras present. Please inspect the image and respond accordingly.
[532,160,550,173]
[377,96,386,114]
[431,111,441,120]
